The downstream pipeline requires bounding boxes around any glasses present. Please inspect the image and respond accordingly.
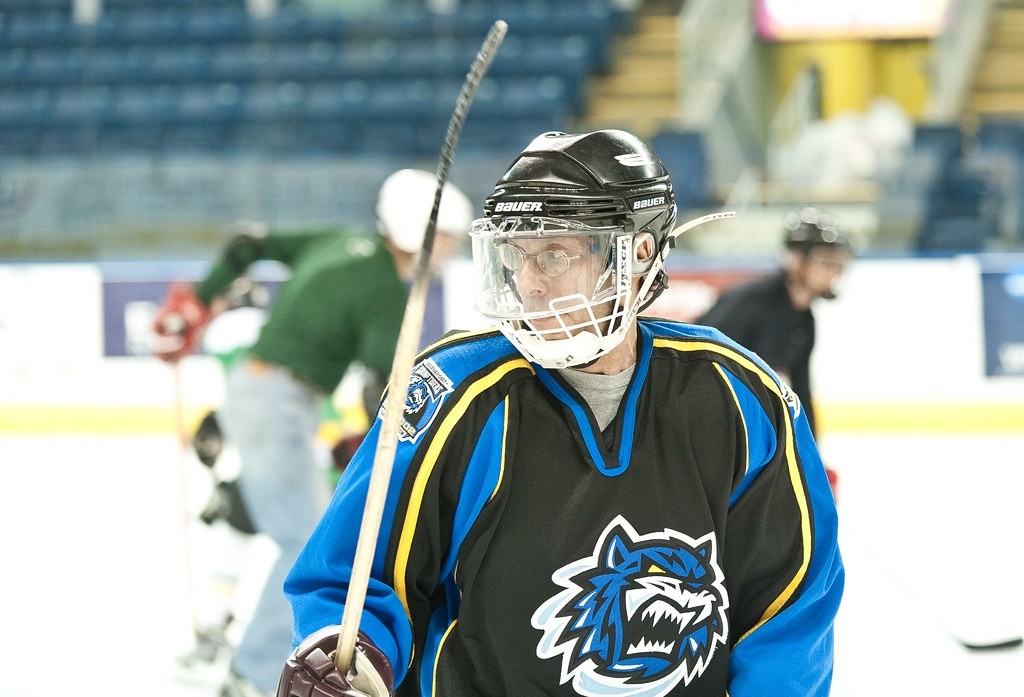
[492,239,601,277]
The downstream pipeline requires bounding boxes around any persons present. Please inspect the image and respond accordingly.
[275,131,845,697]
[692,214,849,502]
[153,167,472,697]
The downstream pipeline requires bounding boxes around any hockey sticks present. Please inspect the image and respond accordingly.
[954,629,1023,654]
[331,20,512,677]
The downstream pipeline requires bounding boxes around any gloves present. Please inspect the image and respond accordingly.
[156,284,213,362]
[276,623,394,697]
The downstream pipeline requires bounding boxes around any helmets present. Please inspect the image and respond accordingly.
[785,205,850,246]
[466,126,678,370]
[373,167,473,254]
